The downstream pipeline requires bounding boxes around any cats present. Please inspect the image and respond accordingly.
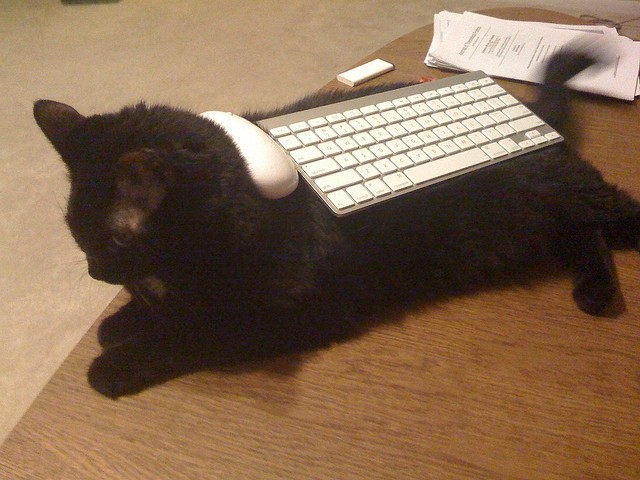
[31,43,640,401]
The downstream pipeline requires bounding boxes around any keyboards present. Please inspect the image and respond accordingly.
[255,70,565,217]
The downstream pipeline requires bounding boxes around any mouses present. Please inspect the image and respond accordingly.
[196,110,299,201]
